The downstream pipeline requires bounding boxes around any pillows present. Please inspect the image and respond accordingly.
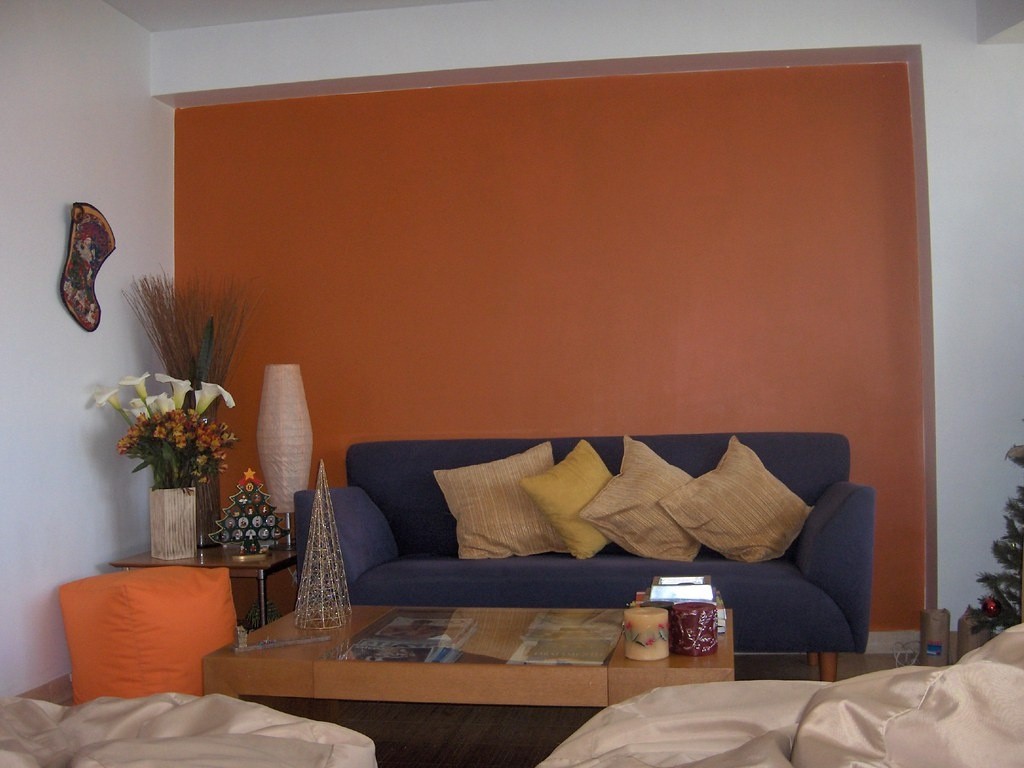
[58,565,239,709]
[578,435,701,561]
[657,435,815,561]
[518,440,614,560]
[432,440,570,560]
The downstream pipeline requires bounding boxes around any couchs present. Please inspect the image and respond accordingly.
[294,431,874,686]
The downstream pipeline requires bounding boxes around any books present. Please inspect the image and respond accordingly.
[336,616,479,663]
[635,575,726,634]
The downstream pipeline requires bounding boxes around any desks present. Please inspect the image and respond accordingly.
[204,603,737,708]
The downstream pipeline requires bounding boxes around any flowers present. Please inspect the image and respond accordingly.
[92,371,240,495]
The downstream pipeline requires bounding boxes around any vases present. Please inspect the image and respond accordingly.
[256,364,322,507]
[148,487,197,560]
